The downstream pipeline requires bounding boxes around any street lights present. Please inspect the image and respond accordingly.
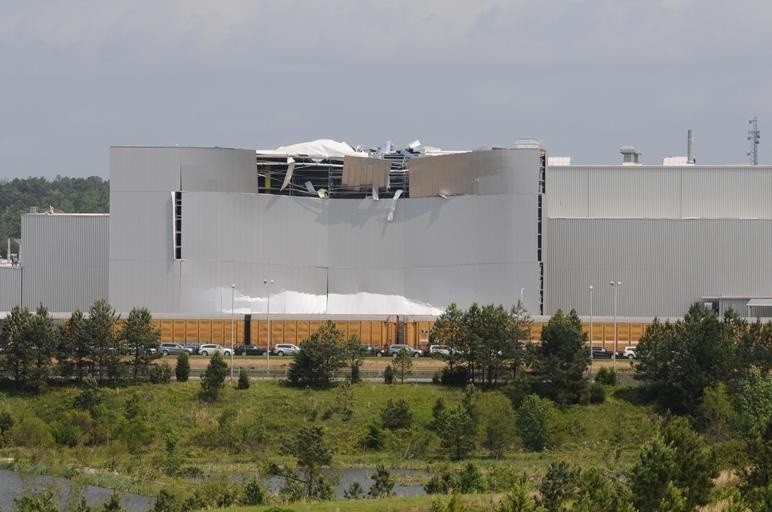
[264,280,274,369]
[610,281,622,360]
[589,285,593,360]
[231,284,235,379]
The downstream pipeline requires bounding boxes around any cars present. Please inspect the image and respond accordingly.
[236,345,272,356]
[146,343,235,355]
[361,345,384,357]
[429,344,462,356]
[587,346,637,359]
[388,344,424,358]
[274,344,300,356]
